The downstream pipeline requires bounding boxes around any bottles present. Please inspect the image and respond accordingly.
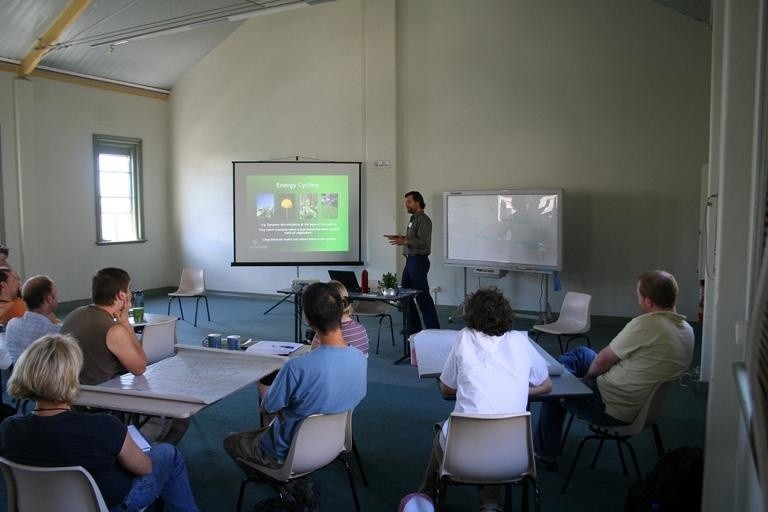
[361,270,368,294]
[132,290,145,324]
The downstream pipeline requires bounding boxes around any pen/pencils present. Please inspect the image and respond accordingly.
[272,345,294,348]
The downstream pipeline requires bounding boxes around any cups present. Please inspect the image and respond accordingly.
[226,335,241,350]
[201,333,222,349]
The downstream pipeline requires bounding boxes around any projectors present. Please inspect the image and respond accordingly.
[291,279,320,290]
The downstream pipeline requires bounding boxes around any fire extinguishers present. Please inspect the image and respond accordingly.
[698,279,705,321]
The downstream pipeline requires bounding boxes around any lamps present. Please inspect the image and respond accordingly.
[92,1,340,48]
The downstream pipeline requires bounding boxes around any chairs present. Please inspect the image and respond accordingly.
[1,456,152,512]
[235,405,357,511]
[419,411,537,512]
[140,316,178,368]
[166,270,211,327]
[350,278,398,355]
[289,275,326,327]
[561,376,678,498]
[550,407,585,457]
[529,291,593,353]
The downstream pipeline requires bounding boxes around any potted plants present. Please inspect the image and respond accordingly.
[377,272,398,298]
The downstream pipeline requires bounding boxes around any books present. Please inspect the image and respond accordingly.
[128,424,152,452]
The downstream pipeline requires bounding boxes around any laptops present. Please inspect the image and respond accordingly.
[329,270,370,292]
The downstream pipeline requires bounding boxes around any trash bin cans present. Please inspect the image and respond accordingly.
[670,366,709,444]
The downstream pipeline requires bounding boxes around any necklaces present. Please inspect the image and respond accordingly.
[32,408,70,412]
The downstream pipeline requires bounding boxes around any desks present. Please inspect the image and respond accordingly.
[5,310,174,366]
[69,341,316,448]
[275,285,423,363]
[436,333,595,504]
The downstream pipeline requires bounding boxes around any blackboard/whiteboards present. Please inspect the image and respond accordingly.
[443,187,563,272]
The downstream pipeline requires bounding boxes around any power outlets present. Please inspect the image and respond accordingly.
[430,287,439,292]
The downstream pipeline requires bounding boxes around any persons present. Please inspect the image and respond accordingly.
[1,334,199,510]
[410,286,552,512]
[223,280,368,511]
[59,267,146,413]
[532,270,695,469]
[383,190,440,336]
[1,247,27,326]
[6,275,64,365]
